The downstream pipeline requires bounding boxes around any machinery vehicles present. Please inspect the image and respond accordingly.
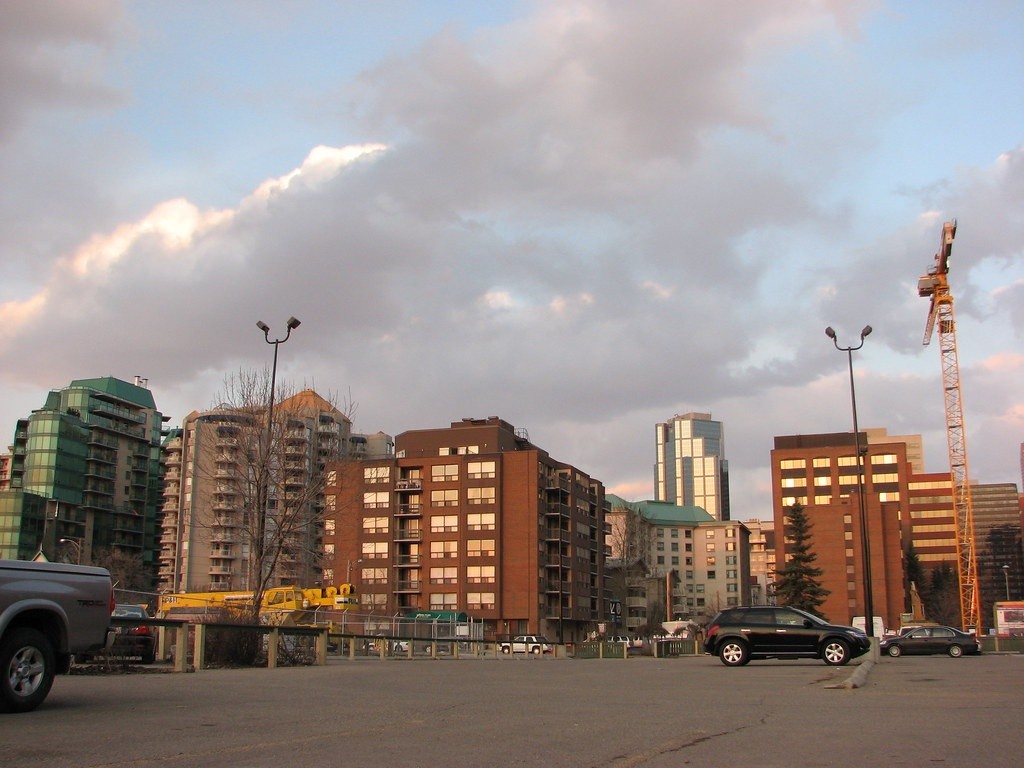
[156,581,358,626]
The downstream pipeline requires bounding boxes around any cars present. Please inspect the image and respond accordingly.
[880,624,982,658]
[423,642,452,654]
[498,635,553,655]
[361,641,413,652]
[605,635,643,651]
[73,603,159,665]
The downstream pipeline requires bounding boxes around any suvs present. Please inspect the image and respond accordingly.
[704,603,872,667]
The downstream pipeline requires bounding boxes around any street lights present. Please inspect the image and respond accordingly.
[60,538,83,565]
[548,472,572,656]
[252,316,302,617]
[1002,565,1010,602]
[822,326,882,662]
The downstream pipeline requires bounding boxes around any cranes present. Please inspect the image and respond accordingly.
[917,219,983,638]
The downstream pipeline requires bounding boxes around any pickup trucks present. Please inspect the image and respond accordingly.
[0,557,116,712]
